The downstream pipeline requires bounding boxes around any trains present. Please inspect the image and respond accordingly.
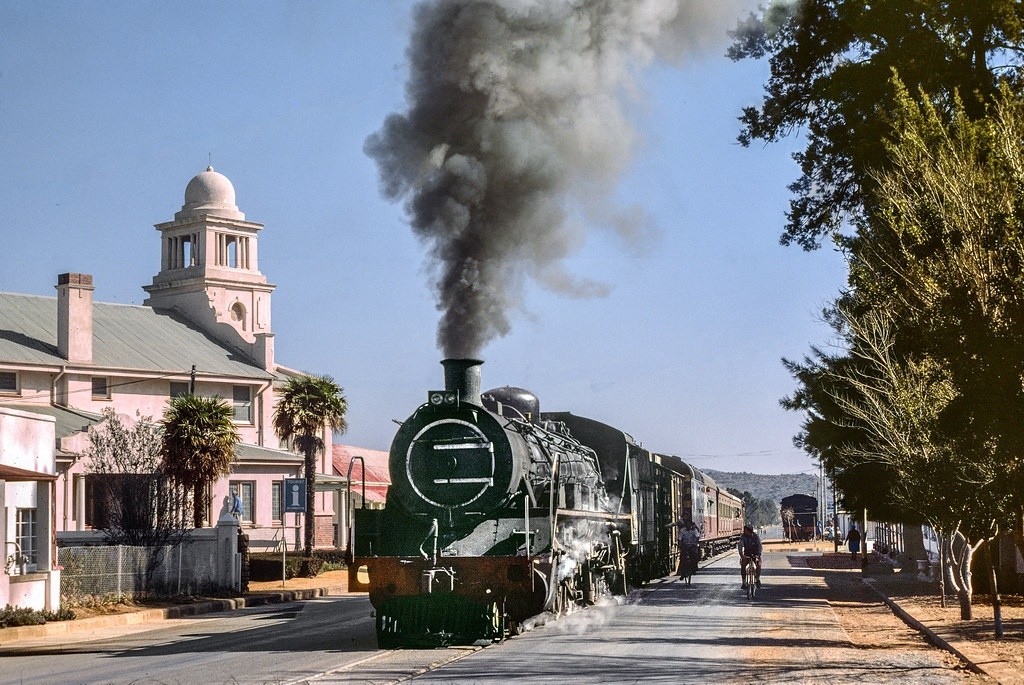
[344,357,748,651]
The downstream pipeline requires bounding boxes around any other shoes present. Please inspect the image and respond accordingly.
[741,582,747,590]
[756,580,762,589]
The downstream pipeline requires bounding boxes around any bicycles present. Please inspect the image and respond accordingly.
[742,553,758,601]
[677,542,697,586]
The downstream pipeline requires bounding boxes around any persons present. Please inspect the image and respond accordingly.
[844,525,861,561]
[676,521,700,581]
[229,490,244,534]
[664,511,701,570]
[738,524,763,590]
